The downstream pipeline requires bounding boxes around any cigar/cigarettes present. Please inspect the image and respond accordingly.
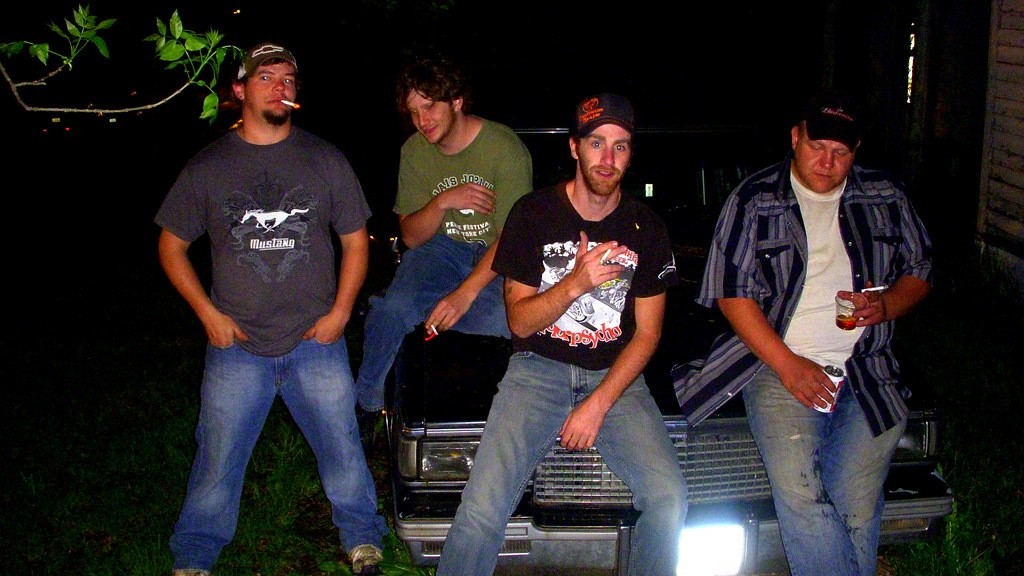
[861,283,888,293]
[600,248,612,264]
[430,324,439,336]
[280,98,299,110]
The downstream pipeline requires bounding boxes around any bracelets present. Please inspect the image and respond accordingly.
[881,295,889,321]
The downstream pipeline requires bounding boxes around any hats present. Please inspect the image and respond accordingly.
[806,104,857,153]
[237,44,298,80]
[574,92,636,136]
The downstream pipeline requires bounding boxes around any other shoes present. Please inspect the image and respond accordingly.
[173,569,211,576]
[349,544,386,576]
[354,402,377,430]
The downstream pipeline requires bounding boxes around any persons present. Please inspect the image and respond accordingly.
[669,103,936,576]
[354,50,534,442]
[435,95,689,576]
[155,41,386,576]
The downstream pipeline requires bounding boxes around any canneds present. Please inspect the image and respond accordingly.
[813,365,845,413]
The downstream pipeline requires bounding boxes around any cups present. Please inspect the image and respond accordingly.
[835,292,868,332]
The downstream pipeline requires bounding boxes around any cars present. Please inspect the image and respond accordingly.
[385,125,957,570]
